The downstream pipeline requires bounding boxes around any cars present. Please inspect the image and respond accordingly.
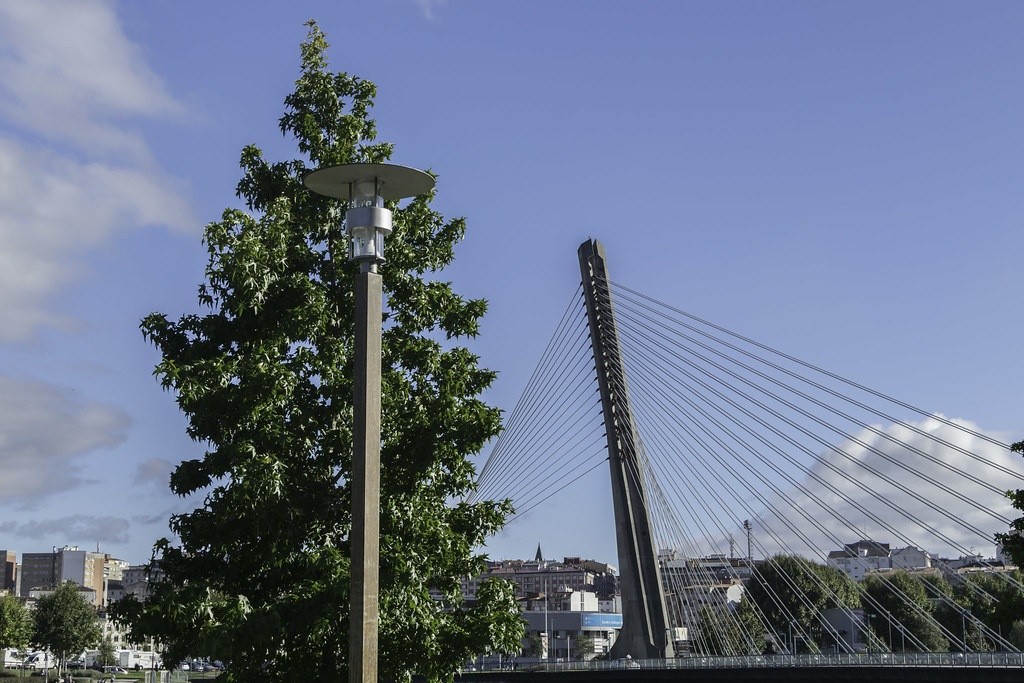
[0,644,227,683]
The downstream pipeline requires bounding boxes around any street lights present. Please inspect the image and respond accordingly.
[301,160,438,683]
